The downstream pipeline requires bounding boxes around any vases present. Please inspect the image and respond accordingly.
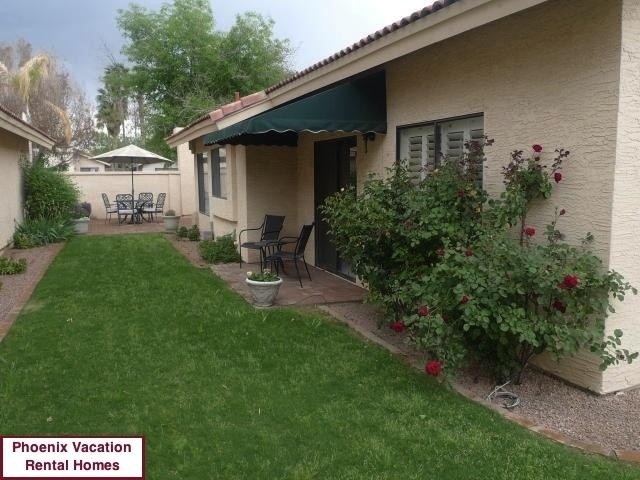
[245,277,284,307]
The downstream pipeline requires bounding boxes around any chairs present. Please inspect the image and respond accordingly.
[141,193,168,225]
[238,211,286,273]
[101,193,120,225]
[115,193,139,226]
[262,223,314,288]
[136,193,154,223]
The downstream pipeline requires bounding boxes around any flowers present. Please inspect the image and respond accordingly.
[245,268,280,282]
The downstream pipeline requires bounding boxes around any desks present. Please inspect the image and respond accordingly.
[114,199,150,224]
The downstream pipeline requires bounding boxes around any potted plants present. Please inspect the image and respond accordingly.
[162,208,181,230]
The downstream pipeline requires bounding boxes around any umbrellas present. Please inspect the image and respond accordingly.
[86,144,172,223]
